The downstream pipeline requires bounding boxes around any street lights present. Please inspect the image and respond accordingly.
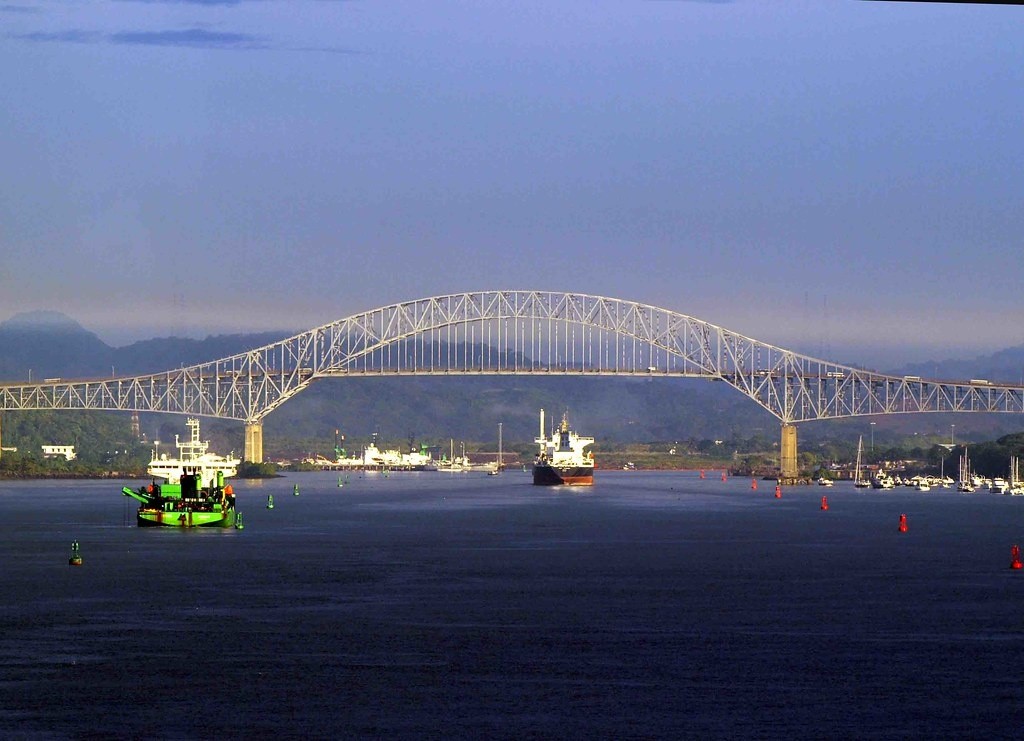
[870,422,877,452]
[28,369,31,385]
[950,424,955,448]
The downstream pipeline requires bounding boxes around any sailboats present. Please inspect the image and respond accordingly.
[435,422,507,476]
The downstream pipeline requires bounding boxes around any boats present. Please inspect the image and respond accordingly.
[119,416,243,528]
[264,441,433,468]
[816,476,834,487]
[852,435,1024,497]
[532,406,597,486]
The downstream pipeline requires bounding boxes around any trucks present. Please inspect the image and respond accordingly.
[970,378,994,386]
[225,370,240,375]
[755,369,774,376]
[326,367,347,374]
[43,377,62,384]
[826,371,845,378]
[903,375,923,381]
